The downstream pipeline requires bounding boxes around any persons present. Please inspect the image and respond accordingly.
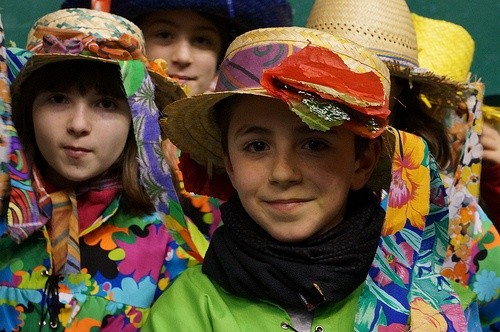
[0,0,500,332]
[137,27,482,332]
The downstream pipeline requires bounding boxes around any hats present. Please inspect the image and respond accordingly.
[155,25,402,178]
[58,0,295,69]
[302,0,479,117]
[411,10,500,120]
[10,9,187,117]
